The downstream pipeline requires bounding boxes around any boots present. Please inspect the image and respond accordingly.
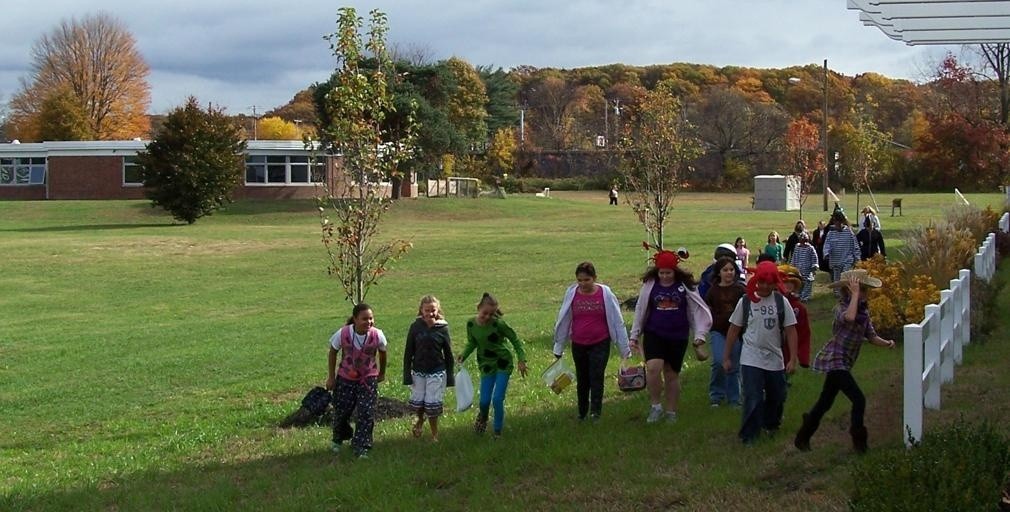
[850,427,870,454]
[796,413,819,451]
[709,399,781,443]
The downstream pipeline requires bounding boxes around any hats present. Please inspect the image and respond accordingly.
[827,269,882,289]
[778,264,802,291]
[655,250,677,269]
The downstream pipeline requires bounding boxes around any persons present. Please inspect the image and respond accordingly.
[551,263,632,421]
[328,304,388,456]
[626,206,896,458]
[456,292,528,439]
[401,297,457,443]
[608,184,618,205]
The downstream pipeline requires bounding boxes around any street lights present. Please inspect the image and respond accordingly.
[788,73,831,213]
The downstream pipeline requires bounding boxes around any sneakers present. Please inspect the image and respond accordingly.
[357,450,368,459]
[647,404,663,425]
[577,413,600,422]
[332,441,341,454]
[412,426,439,443]
[665,410,676,423]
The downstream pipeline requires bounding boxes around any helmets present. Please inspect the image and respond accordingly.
[712,244,737,264]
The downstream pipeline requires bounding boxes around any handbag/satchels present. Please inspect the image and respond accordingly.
[618,346,647,392]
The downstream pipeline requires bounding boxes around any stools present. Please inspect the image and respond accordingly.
[890,198,903,216]
